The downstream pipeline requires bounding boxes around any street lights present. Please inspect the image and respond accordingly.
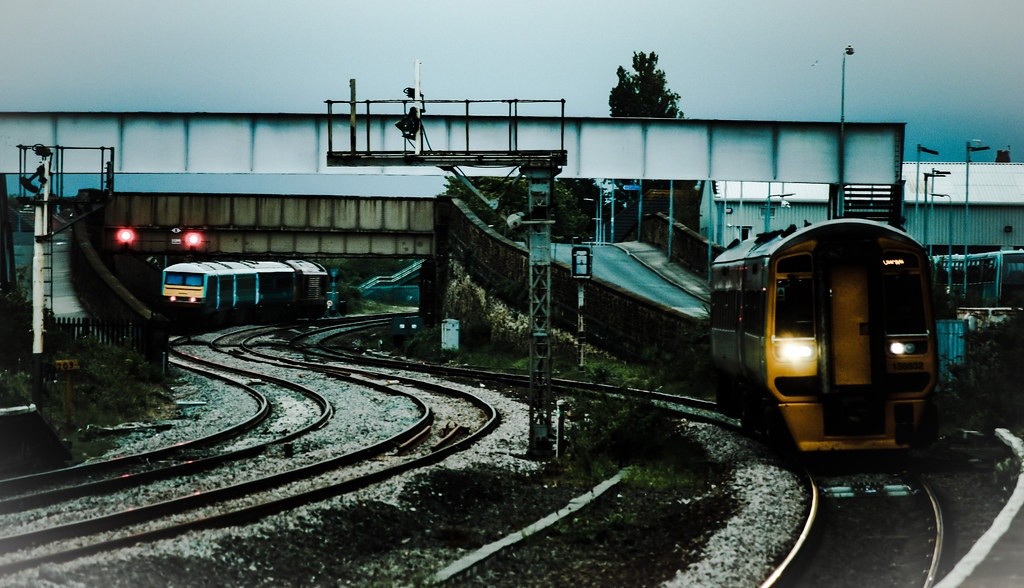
[915,144,951,255]
[835,46,856,218]
[963,141,990,299]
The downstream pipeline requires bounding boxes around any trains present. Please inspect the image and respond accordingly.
[708,217,940,471]
[160,259,329,332]
